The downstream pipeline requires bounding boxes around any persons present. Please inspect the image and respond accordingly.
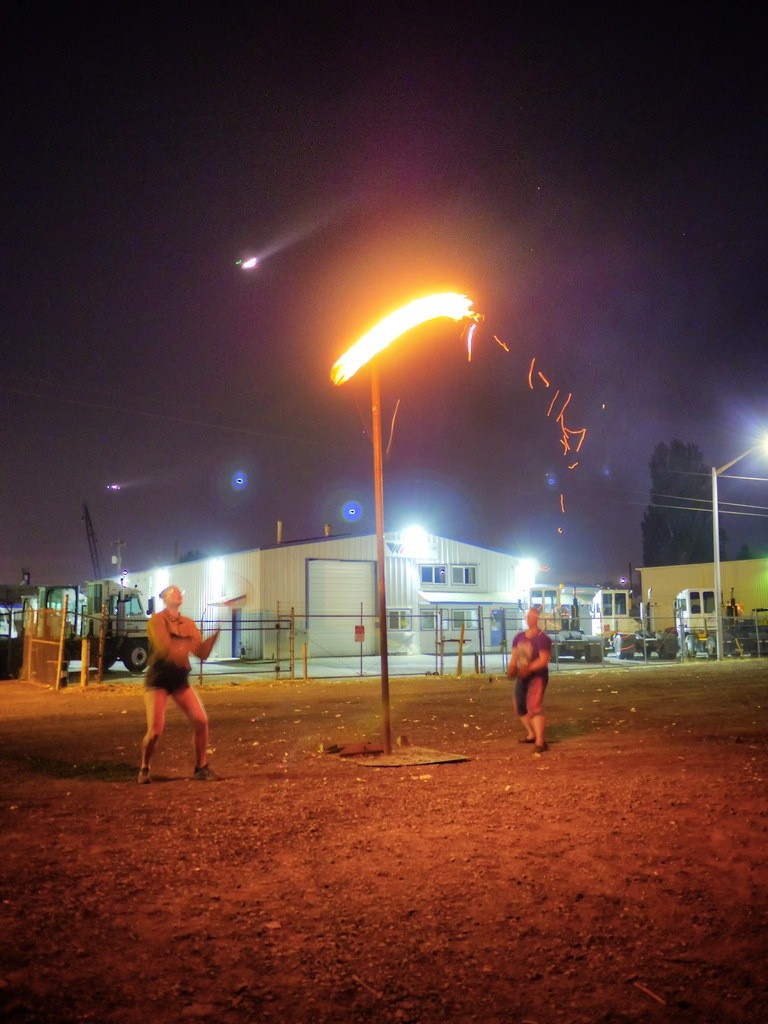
[137,585,223,785]
[503,610,553,753]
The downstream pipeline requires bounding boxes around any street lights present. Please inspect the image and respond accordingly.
[711,435,768,661]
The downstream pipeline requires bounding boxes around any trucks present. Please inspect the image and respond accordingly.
[590,589,680,661]
[673,588,768,657]
[515,586,603,664]
[1,577,157,684]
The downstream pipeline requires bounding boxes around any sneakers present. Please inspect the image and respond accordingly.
[193,764,219,781]
[136,768,152,784]
[518,736,537,743]
[532,740,548,754]
